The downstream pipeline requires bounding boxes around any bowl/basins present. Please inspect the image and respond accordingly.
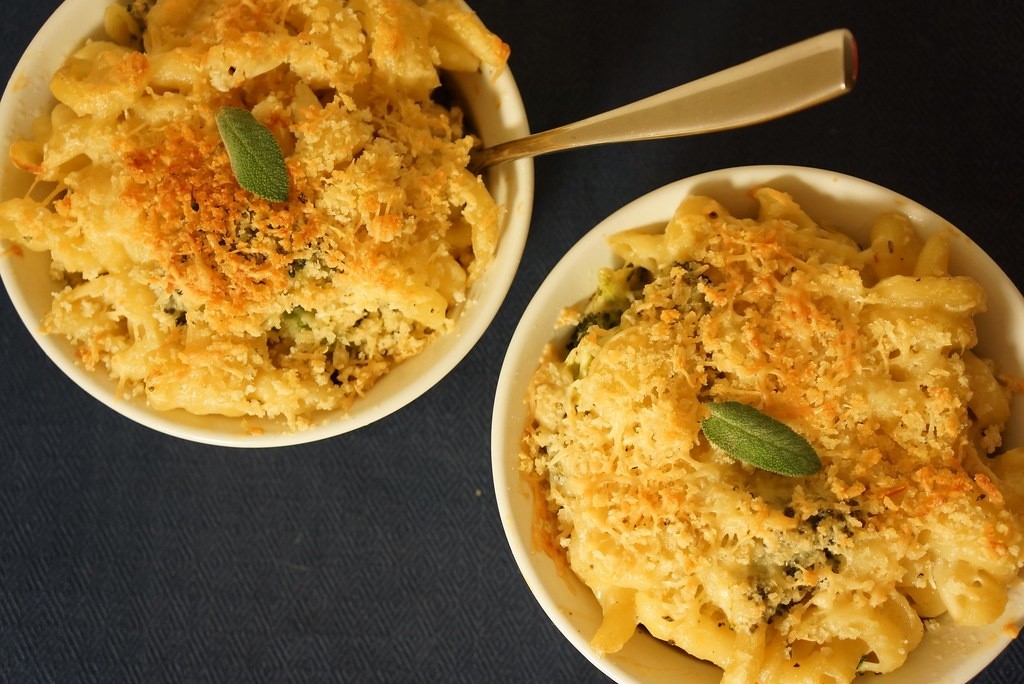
[0,0,533,451]
[489,166,1024,684]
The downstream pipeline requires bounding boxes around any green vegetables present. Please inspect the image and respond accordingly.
[214,106,290,204]
[700,402,822,478]
